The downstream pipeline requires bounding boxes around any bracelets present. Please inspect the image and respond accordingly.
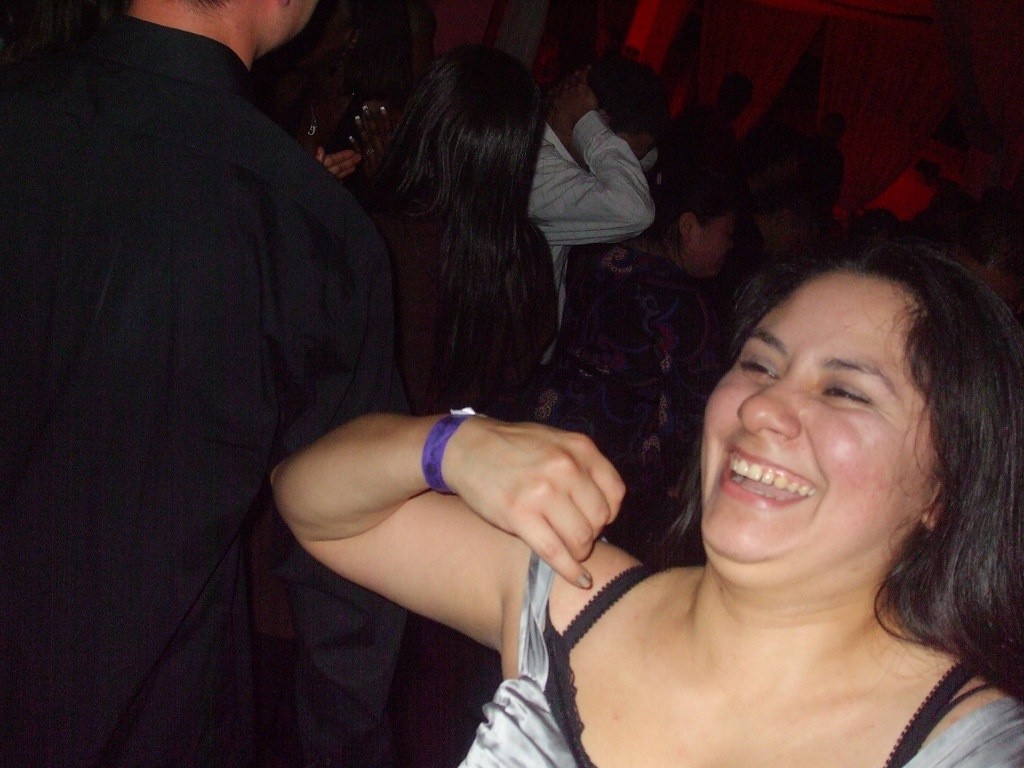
[420,405,487,496]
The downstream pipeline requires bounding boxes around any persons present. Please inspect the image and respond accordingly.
[272,243,1024,764]
[3,0,1024,766]
